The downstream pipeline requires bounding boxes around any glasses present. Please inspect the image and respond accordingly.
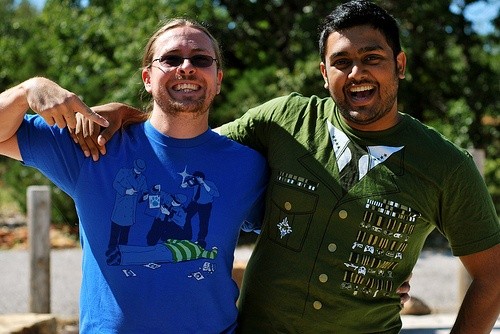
[148,55,220,70]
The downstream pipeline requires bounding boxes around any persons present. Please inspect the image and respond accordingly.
[67,1,500,334]
[1,15,272,334]
